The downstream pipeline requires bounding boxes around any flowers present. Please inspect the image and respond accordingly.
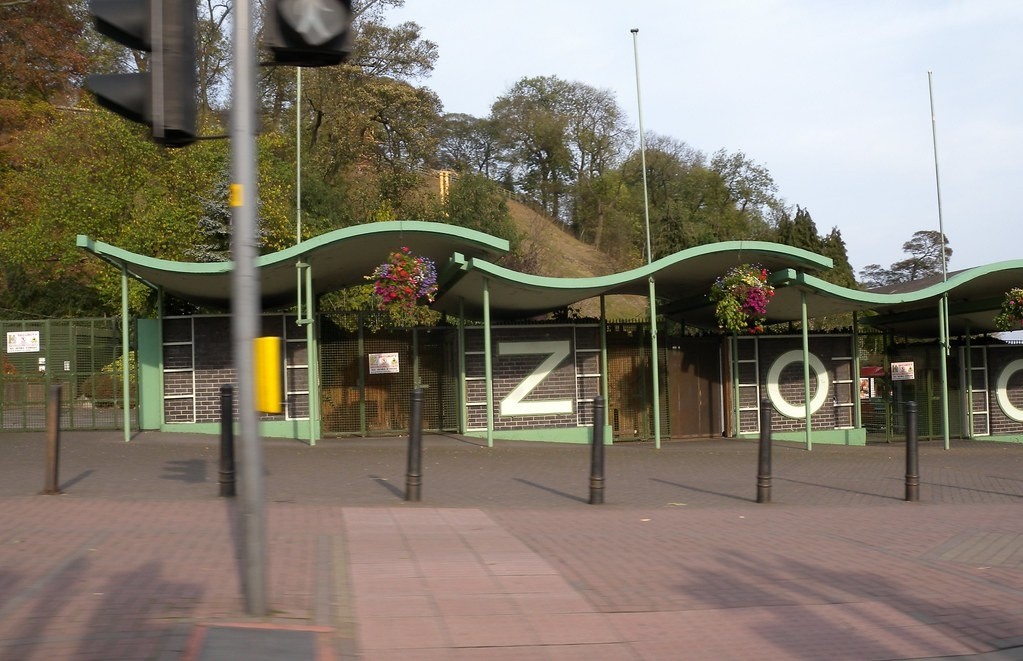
[993,289,1023,330]
[710,263,777,337]
[362,247,440,309]
